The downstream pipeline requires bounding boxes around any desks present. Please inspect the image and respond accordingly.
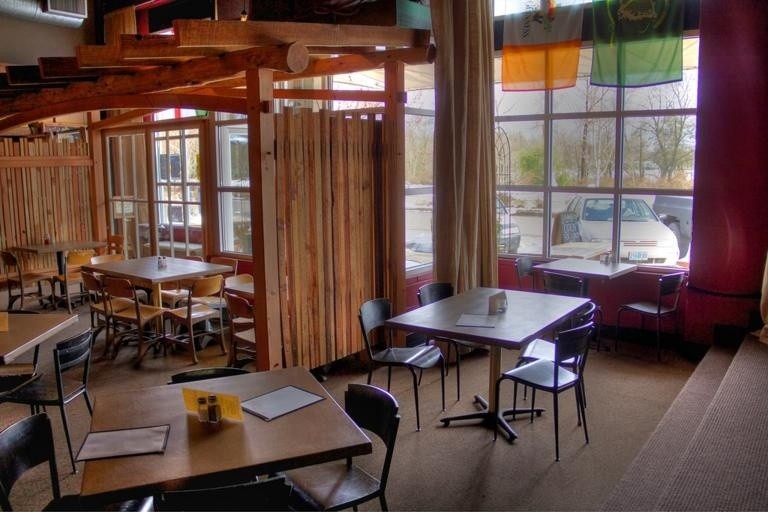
[386,287,591,441]
[532,258,638,351]
[79,365,372,511]
[13,239,105,309]
[1,315,81,365]
[546,241,624,260]
[224,283,254,299]
[79,254,232,351]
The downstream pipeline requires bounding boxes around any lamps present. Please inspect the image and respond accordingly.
[241,0,248,22]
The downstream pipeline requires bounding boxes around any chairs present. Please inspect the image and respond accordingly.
[90,254,147,304]
[357,297,446,432]
[53,248,95,315]
[543,272,584,297]
[170,368,250,384]
[416,282,492,401]
[163,274,223,362]
[1,312,43,420]
[1,251,56,314]
[224,293,258,365]
[3,326,93,476]
[106,277,164,362]
[2,413,155,509]
[509,303,595,427]
[514,256,538,290]
[494,322,593,464]
[615,270,685,362]
[106,239,123,255]
[81,270,138,353]
[180,258,238,335]
[225,273,255,330]
[282,384,401,509]
[162,256,204,333]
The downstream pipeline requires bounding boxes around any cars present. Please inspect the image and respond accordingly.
[404,205,431,254]
[166,188,201,223]
[563,193,680,265]
[495,194,520,256]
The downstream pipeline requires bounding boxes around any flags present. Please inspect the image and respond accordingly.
[501,0,584,92]
[589,0,685,88]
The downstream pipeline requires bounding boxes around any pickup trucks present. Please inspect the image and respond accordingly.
[652,195,693,260]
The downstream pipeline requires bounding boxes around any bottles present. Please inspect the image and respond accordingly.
[157,256,163,267]
[162,256,168,268]
[44,234,50,246]
[197,396,209,422]
[207,394,222,423]
[605,253,610,265]
